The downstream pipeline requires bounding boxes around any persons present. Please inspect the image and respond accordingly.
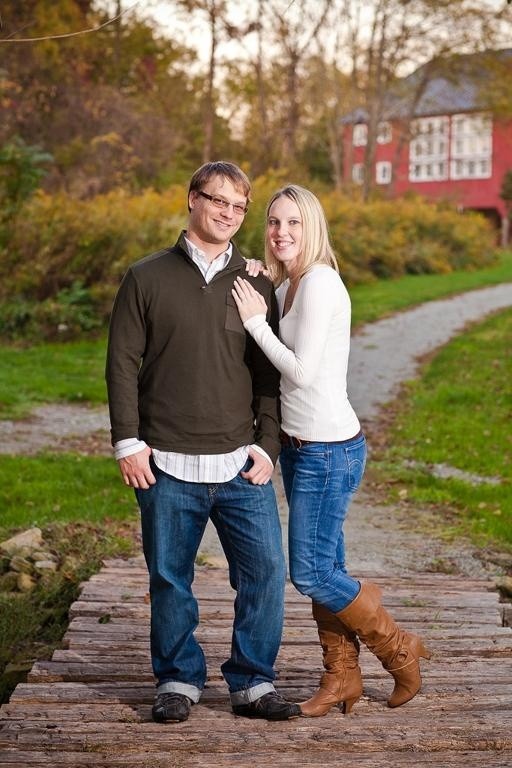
[232,185,433,717]
[105,157,301,722]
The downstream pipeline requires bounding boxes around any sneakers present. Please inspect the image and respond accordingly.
[152,693,190,723]
[232,690,302,720]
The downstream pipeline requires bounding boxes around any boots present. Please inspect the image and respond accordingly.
[294,591,364,719]
[336,580,432,708]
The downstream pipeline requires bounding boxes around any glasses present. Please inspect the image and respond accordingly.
[198,190,250,216]
